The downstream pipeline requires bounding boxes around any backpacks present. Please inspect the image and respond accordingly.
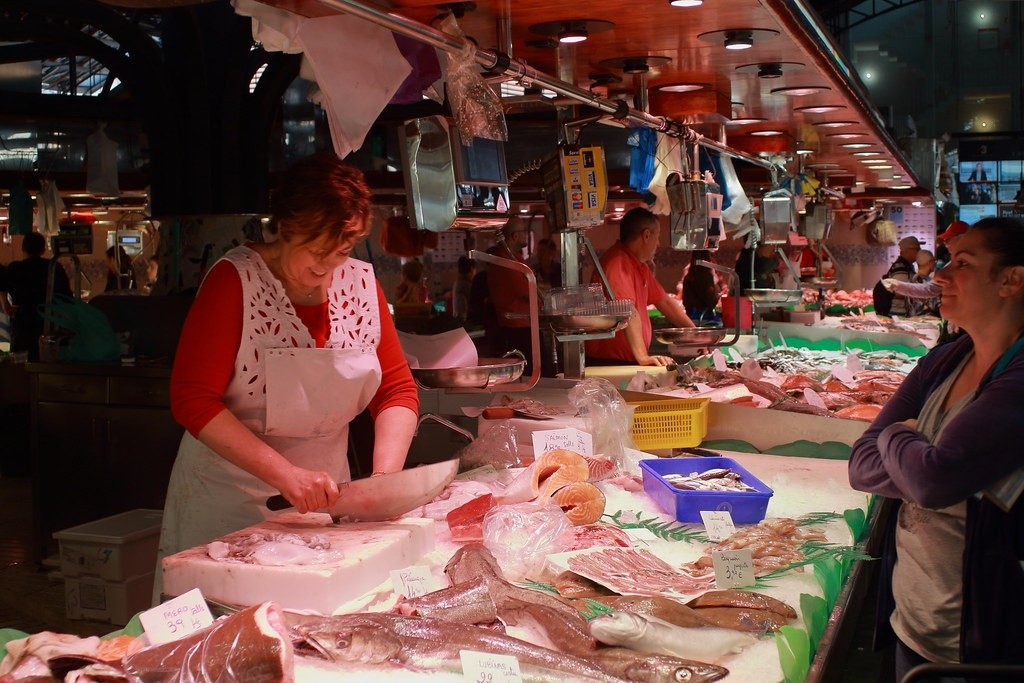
[872,261,911,317]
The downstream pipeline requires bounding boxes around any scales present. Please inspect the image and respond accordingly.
[652,180,741,348]
[743,188,802,307]
[798,187,842,289]
[38,224,93,360]
[397,56,541,394]
[115,230,146,291]
[540,145,632,342]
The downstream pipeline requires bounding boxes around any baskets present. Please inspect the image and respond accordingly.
[626,396,711,451]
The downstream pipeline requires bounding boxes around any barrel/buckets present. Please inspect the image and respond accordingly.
[721,297,752,328]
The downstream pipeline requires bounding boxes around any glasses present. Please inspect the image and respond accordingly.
[910,247,921,252]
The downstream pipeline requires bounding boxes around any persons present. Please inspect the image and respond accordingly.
[151,152,420,610]
[486,217,551,376]
[104,245,137,293]
[886,236,951,319]
[452,256,478,331]
[531,239,562,288]
[730,220,800,297]
[968,163,986,181]
[882,221,969,344]
[683,250,728,326]
[469,246,504,358]
[396,261,423,304]
[0,232,73,363]
[848,215,1024,683]
[584,207,696,366]
[970,188,991,203]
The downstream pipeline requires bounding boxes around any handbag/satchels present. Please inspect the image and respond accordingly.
[38,295,123,364]
[868,217,897,246]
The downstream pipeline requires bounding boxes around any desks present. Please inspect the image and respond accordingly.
[676,290,944,448]
[27,448,893,683]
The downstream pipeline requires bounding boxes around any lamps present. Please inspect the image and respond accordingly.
[758,64,782,79]
[560,25,587,43]
[623,62,649,75]
[724,34,753,50]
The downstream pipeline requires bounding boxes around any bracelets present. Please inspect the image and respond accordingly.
[371,471,386,476]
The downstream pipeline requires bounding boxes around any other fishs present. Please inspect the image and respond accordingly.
[840,319,939,340]
[688,591,798,636]
[758,347,921,373]
[698,369,904,424]
[293,543,733,683]
[663,468,758,492]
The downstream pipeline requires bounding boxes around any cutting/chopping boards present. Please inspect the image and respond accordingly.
[585,365,668,387]
[163,511,435,615]
[477,408,593,456]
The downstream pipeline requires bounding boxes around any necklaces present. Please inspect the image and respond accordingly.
[296,289,316,297]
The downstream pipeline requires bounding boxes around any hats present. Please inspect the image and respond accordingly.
[916,250,935,264]
[899,237,927,246]
[937,221,968,239]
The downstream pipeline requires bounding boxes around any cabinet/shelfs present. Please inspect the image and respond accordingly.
[24,363,184,537]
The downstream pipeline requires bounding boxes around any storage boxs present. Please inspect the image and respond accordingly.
[52,506,162,627]
[638,456,773,523]
